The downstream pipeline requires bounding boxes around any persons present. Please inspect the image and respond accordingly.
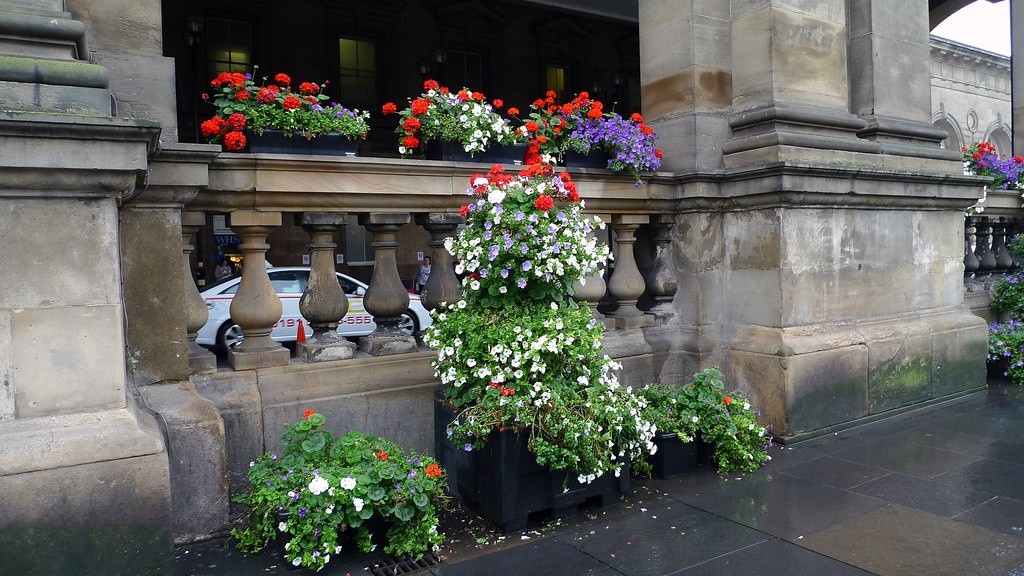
[235,258,244,276]
[452,260,466,284]
[214,258,233,281]
[416,255,433,296]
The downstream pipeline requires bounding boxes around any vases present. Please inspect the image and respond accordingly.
[648,434,715,474]
[434,391,631,533]
[990,355,1012,382]
[249,129,359,156]
[272,510,391,571]
[425,136,526,165]
[565,148,609,168]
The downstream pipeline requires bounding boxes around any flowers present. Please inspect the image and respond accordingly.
[635,366,773,475]
[421,159,658,495]
[526,91,662,188]
[229,410,449,574]
[961,141,1024,199]
[381,78,532,161]
[987,234,1024,385]
[200,64,372,152]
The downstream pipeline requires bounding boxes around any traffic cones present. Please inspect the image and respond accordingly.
[294,319,308,358]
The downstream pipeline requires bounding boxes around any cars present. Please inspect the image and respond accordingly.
[194,266,434,354]
